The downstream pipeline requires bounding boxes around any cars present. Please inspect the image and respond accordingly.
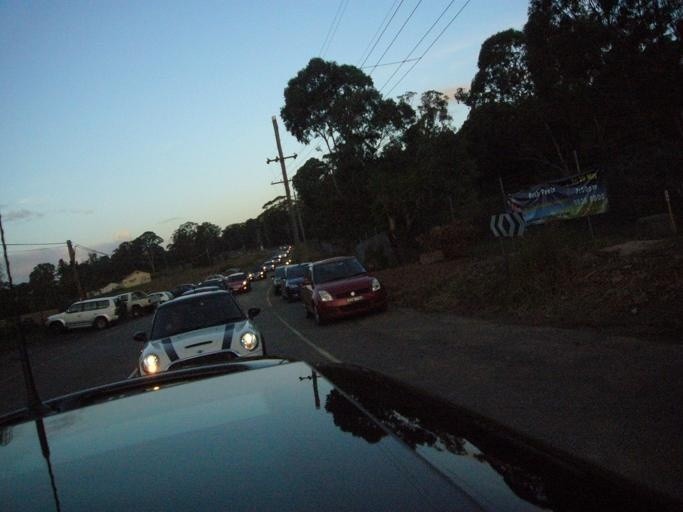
[130,288,266,379]
[0,355,681,512]
[113,243,391,327]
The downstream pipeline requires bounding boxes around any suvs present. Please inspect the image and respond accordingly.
[44,296,127,338]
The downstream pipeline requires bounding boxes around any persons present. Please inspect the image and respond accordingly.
[113,297,128,324]
